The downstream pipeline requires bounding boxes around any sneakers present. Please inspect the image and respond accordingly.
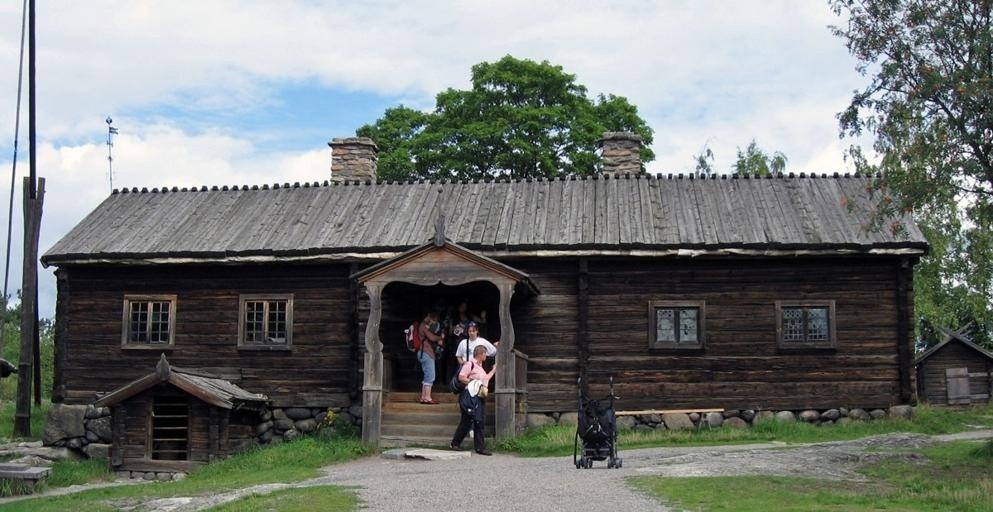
[421,398,439,404]
[449,442,462,451]
[476,448,492,456]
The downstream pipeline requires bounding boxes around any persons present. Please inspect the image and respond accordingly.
[415,297,500,456]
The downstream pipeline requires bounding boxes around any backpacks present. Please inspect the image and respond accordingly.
[405,321,426,352]
[583,400,610,440]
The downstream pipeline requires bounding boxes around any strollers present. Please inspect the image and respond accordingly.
[572,374,623,470]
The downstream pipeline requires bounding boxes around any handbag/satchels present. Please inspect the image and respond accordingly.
[450,361,474,394]
